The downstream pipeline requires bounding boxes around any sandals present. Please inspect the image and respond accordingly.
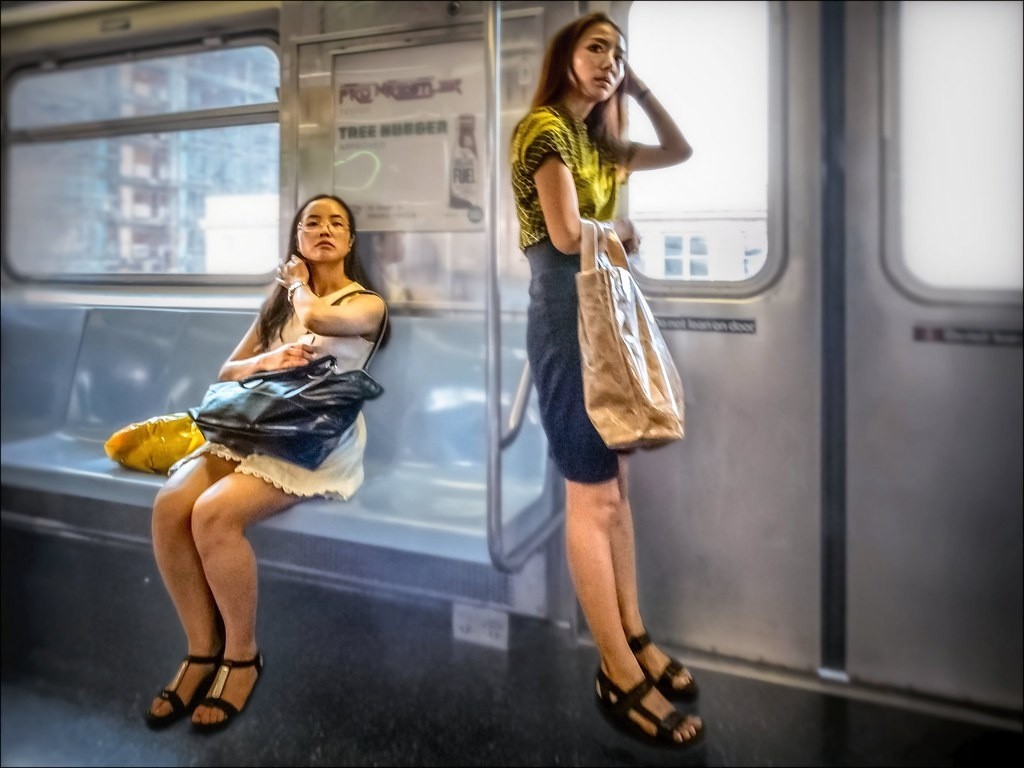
[191,648,266,734]
[630,628,700,705]
[143,649,225,730]
[594,663,706,749]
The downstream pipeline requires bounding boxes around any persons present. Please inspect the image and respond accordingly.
[145,190,391,733]
[508,11,706,750]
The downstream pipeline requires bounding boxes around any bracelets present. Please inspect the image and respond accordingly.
[636,88,650,104]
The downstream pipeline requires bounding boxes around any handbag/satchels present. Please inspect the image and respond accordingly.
[194,289,389,470]
[577,218,686,452]
[105,413,207,475]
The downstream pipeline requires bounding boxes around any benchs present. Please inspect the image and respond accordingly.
[0,302,583,651]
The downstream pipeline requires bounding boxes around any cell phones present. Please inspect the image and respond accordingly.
[299,335,316,347]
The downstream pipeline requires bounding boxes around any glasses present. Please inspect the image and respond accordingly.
[296,218,352,233]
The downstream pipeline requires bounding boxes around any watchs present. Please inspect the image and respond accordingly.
[288,281,310,306]
